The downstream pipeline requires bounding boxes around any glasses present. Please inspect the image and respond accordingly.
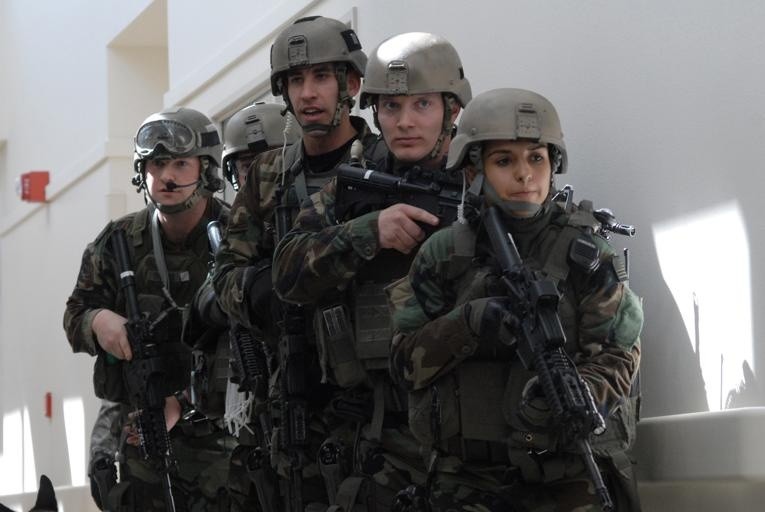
[133,119,220,158]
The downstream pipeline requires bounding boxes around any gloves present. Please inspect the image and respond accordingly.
[518,377,552,429]
[470,295,521,351]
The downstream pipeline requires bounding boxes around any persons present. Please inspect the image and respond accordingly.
[271,33,472,512]
[383,89,642,511]
[212,17,380,509]
[61,102,301,512]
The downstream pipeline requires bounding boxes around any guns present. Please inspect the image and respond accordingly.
[473,206,615,511]
[103,228,179,512]
[262,203,308,511]
[204,220,276,455]
[336,164,636,279]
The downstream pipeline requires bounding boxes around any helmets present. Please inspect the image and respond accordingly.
[358,31,472,110]
[222,103,303,191]
[270,15,367,96]
[134,107,222,173]
[443,87,568,185]
